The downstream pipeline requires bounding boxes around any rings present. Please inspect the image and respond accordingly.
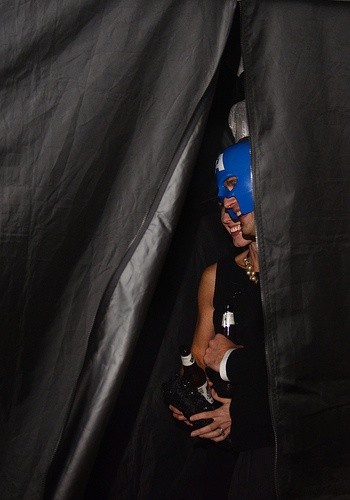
[219,428,223,433]
[222,433,225,436]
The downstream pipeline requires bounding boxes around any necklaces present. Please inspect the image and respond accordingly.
[243,252,259,285]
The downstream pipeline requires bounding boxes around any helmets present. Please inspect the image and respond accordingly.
[215,140,256,221]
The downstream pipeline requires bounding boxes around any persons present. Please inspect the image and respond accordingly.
[167,139,273,499]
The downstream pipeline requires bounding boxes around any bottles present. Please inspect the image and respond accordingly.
[180,350,217,412]
